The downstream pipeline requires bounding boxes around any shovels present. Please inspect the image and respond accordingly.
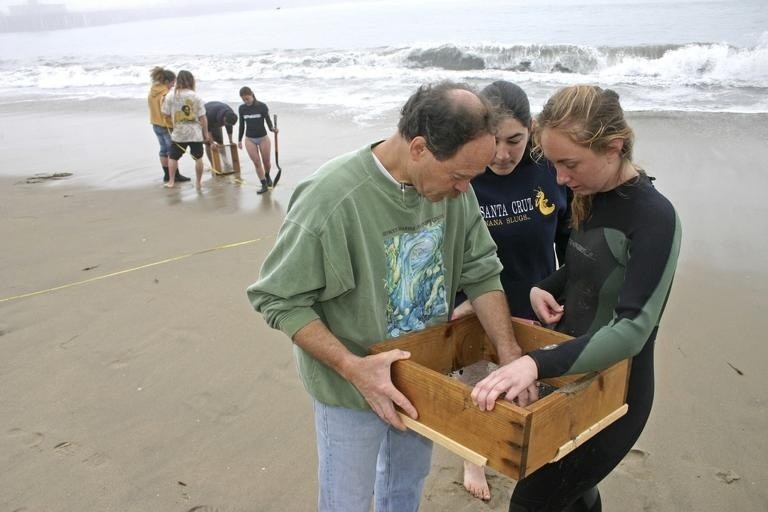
[273,114,281,188]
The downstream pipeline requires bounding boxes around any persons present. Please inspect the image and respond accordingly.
[202,100,238,168]
[452,81,574,501]
[246,78,541,512]
[160,69,211,191]
[147,65,192,182]
[469,82,684,512]
[238,86,279,195]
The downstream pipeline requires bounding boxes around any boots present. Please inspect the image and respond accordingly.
[162,166,190,181]
[265,172,272,186]
[257,180,268,194]
